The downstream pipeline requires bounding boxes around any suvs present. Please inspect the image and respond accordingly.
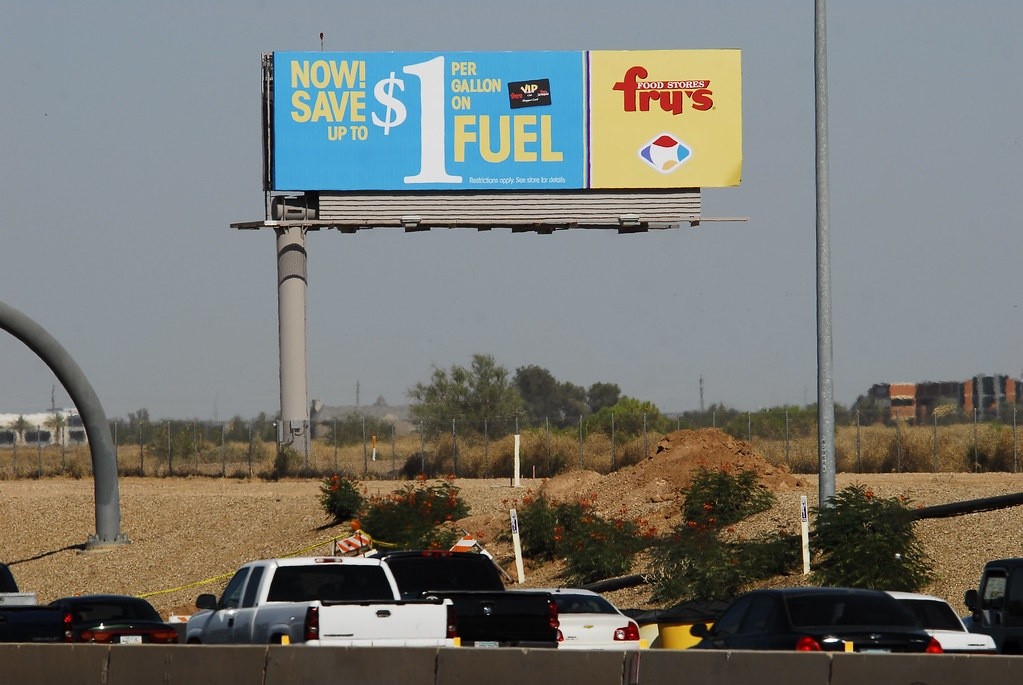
[961,555,1022,656]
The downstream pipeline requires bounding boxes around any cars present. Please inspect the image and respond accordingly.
[30,593,180,644]
[507,587,641,648]
[686,587,945,653]
[884,590,997,652]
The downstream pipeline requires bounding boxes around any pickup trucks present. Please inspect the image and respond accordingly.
[179,557,462,649]
[370,550,560,649]
